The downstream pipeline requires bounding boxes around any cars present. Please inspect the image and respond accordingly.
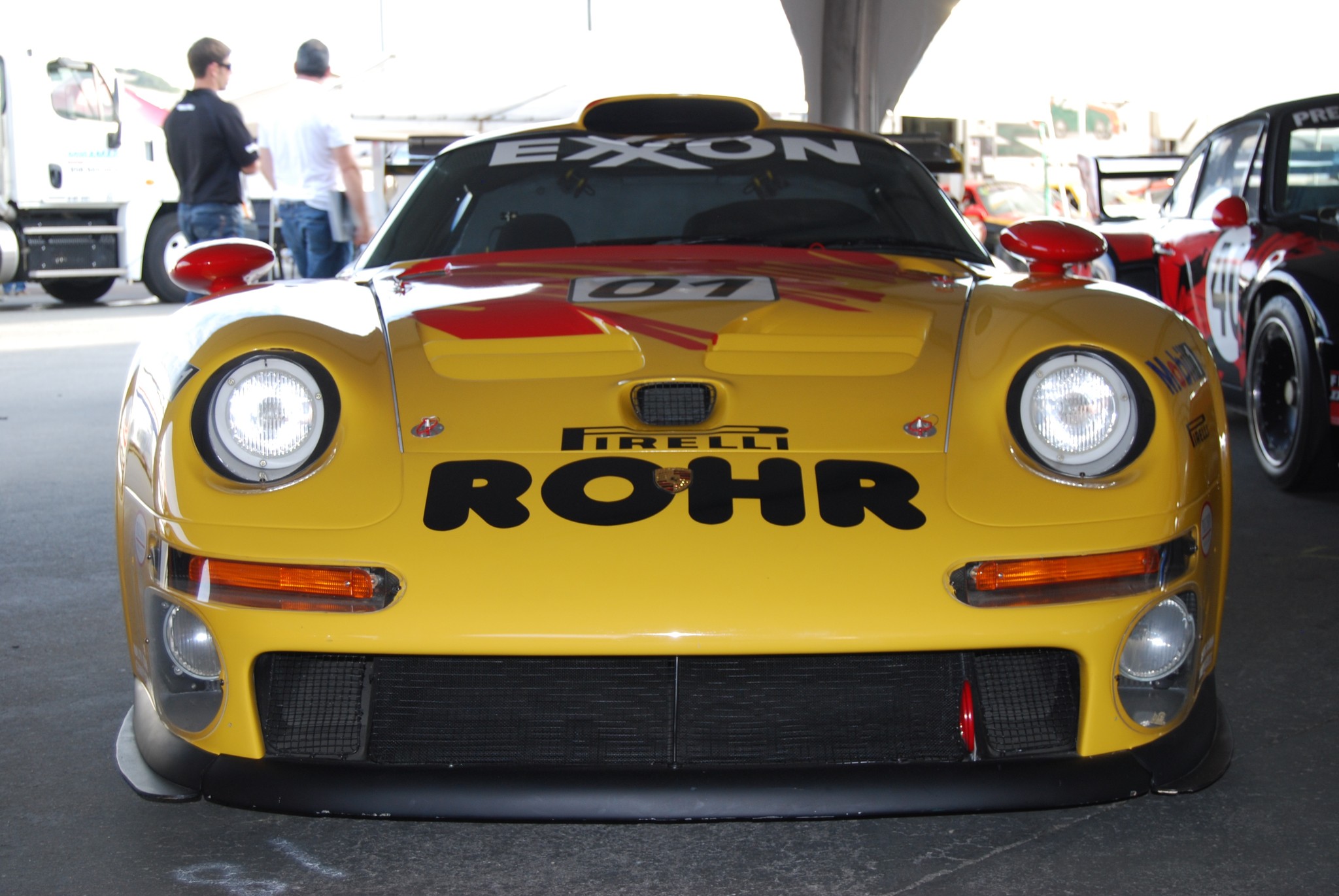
[1159,96,1338,478]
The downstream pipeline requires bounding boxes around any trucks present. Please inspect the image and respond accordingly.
[5,50,274,301]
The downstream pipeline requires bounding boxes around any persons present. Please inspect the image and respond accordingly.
[164,37,263,306]
[256,38,375,281]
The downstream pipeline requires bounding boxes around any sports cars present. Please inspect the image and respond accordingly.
[109,80,1239,827]
